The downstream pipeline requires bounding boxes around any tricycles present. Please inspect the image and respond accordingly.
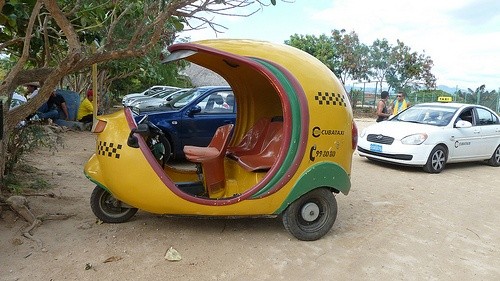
[84,39,358,241]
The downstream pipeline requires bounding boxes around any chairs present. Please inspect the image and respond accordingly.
[184,124,235,192]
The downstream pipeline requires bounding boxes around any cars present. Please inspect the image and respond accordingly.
[120,84,238,167]
[356,97,500,175]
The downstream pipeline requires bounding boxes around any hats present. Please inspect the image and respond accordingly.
[25,82,40,87]
[87,89,93,96]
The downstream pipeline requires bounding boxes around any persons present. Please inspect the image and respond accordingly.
[76,89,101,122]
[388,92,413,115]
[23,81,69,124]
[375,91,391,123]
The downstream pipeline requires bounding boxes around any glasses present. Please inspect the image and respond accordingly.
[397,95,402,97]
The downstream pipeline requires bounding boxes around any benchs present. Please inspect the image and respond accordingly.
[225,116,285,195]
